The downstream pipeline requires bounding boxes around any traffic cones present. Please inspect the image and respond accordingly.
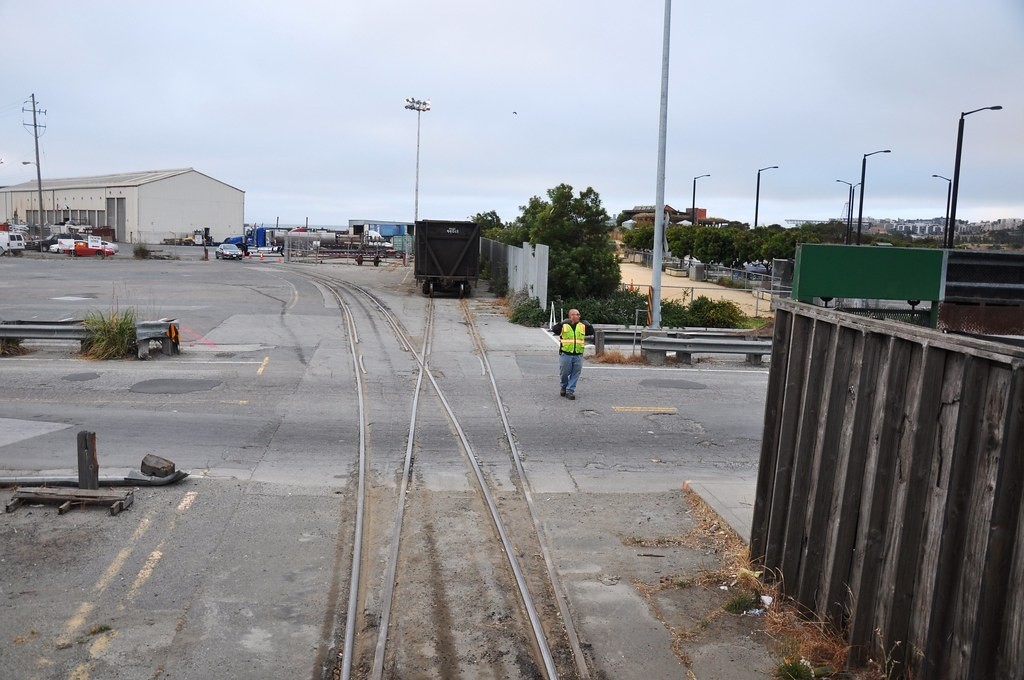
[260,253,263,260]
[630,278,635,291]
[248,251,252,259]
[241,251,246,260]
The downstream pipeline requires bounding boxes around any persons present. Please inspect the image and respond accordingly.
[552,309,594,400]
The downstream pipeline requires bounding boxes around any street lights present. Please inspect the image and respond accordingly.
[932,174,952,246]
[692,174,710,227]
[949,106,1003,250]
[834,180,862,245]
[22,160,45,254]
[404,97,432,220]
[856,149,891,246]
[754,166,779,229]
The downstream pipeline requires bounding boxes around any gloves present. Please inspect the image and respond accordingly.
[563,319,572,324]
[582,320,590,327]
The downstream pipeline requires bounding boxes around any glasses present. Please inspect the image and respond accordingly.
[572,314,581,316]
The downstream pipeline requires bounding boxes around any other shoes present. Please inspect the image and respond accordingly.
[560,387,566,396]
[565,394,575,400]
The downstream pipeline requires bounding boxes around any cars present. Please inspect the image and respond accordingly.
[684,256,700,265]
[744,262,772,280]
[215,244,243,260]
[0,221,119,257]
[709,259,725,271]
